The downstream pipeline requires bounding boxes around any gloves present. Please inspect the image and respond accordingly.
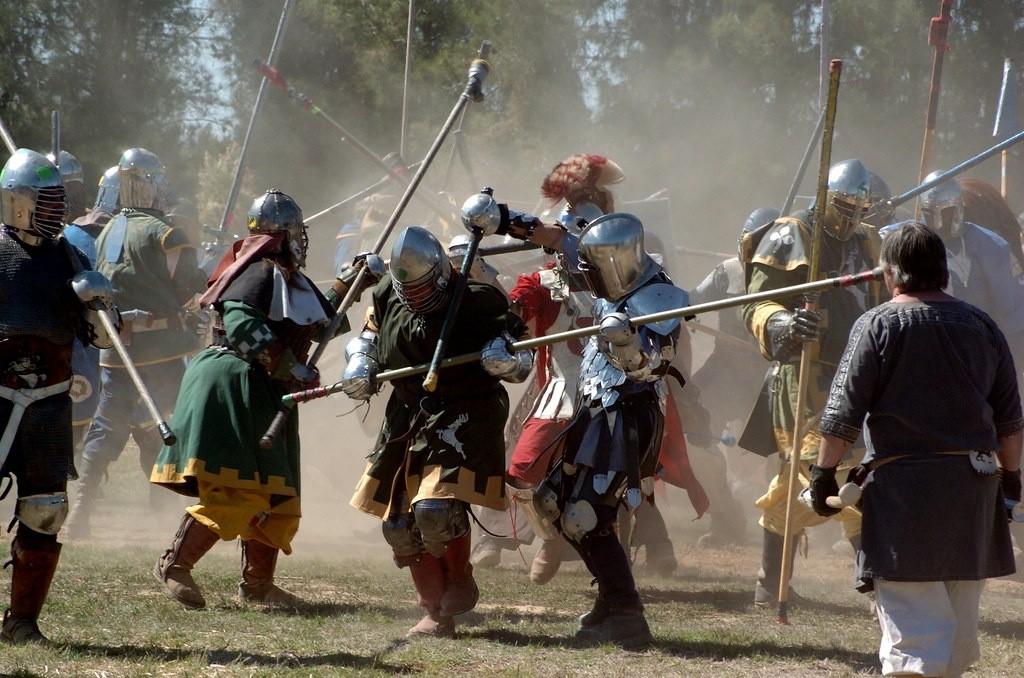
[600,311,642,361]
[70,270,118,326]
[195,312,211,339]
[331,251,385,307]
[271,346,321,394]
[766,308,825,363]
[999,467,1022,524]
[808,462,844,517]
[343,337,384,400]
[198,241,231,278]
[480,331,532,383]
[460,194,509,236]
[448,234,500,285]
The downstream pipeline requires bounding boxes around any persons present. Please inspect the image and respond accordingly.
[682,159,1023,613]
[809,221,1024,677]
[0,148,122,648]
[40,148,709,644]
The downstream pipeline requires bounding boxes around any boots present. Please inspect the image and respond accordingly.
[0,455,877,649]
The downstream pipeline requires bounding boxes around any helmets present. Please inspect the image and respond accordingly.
[119,148,168,212]
[576,212,664,303]
[737,161,967,272]
[389,226,452,315]
[539,153,626,277]
[247,187,310,271]
[167,198,200,226]
[0,148,70,241]
[93,166,120,213]
[45,148,84,185]
